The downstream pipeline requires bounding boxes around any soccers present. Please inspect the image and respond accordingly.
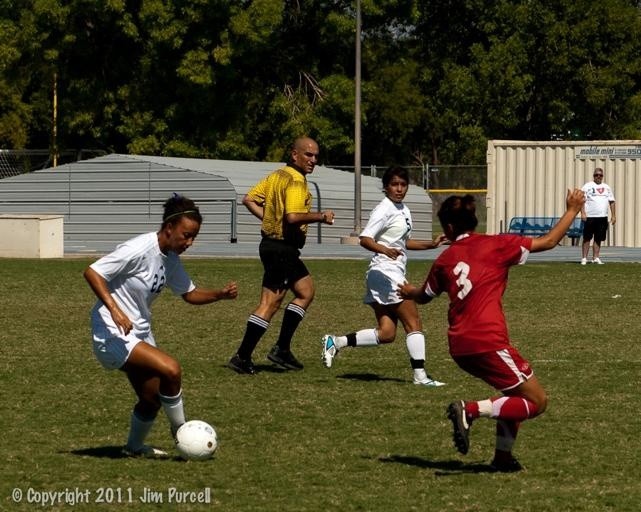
[175,419,218,461]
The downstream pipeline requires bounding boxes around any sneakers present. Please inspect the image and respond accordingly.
[413,376,446,387]
[581,258,587,265]
[228,352,257,374]
[490,454,523,473]
[268,344,304,370]
[592,257,604,265]
[447,398,473,455]
[120,444,168,458]
[321,334,339,369]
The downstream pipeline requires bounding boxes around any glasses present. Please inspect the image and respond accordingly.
[593,174,603,177]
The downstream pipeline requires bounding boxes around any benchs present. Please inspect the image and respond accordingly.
[506,218,585,246]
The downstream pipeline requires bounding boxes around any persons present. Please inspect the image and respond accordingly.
[228,133,334,374]
[321,166,451,387]
[83,195,239,457]
[579,168,616,265]
[396,188,585,472]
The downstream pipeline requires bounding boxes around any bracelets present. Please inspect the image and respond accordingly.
[321,213,328,221]
